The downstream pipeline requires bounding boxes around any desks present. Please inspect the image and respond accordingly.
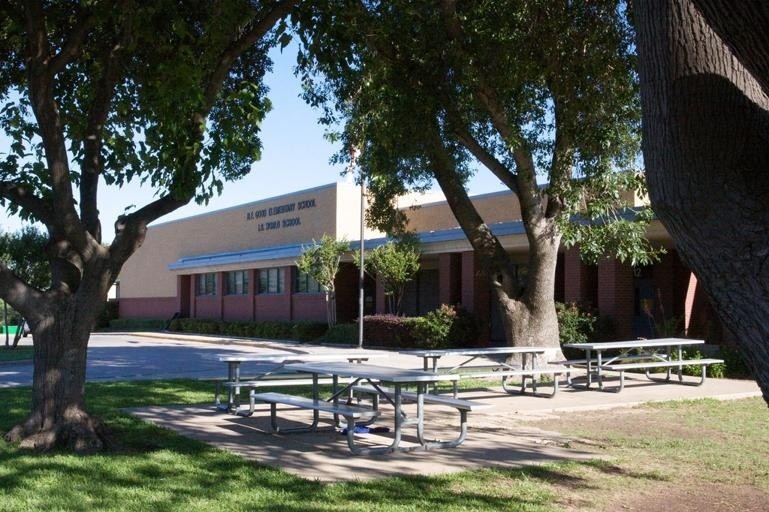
[285,361,459,453]
[398,346,562,399]
[563,337,706,393]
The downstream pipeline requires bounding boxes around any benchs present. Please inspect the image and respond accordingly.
[350,384,494,450]
[215,353,725,416]
[250,393,381,454]
